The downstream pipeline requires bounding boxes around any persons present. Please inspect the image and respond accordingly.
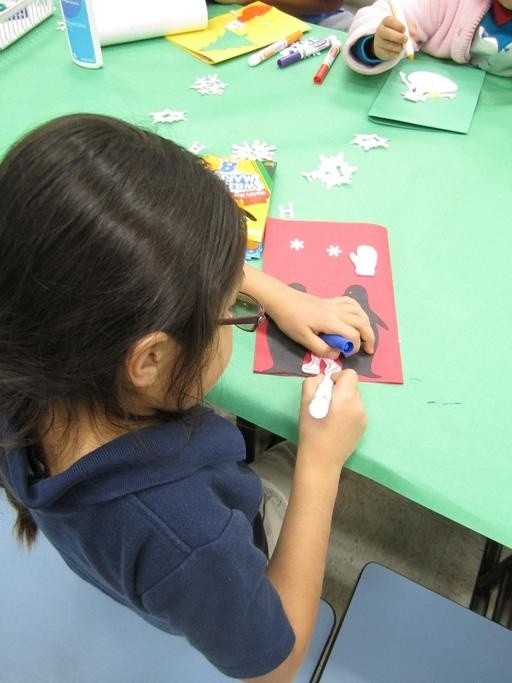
[341,0,512,79]
[0,109,376,682]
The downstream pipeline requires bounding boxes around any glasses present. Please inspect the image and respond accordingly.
[217,291,264,332]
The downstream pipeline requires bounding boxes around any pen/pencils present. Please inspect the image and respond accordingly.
[392,8,415,63]
[313,46,340,83]
[276,38,329,65]
[306,376,335,420]
[246,31,307,66]
